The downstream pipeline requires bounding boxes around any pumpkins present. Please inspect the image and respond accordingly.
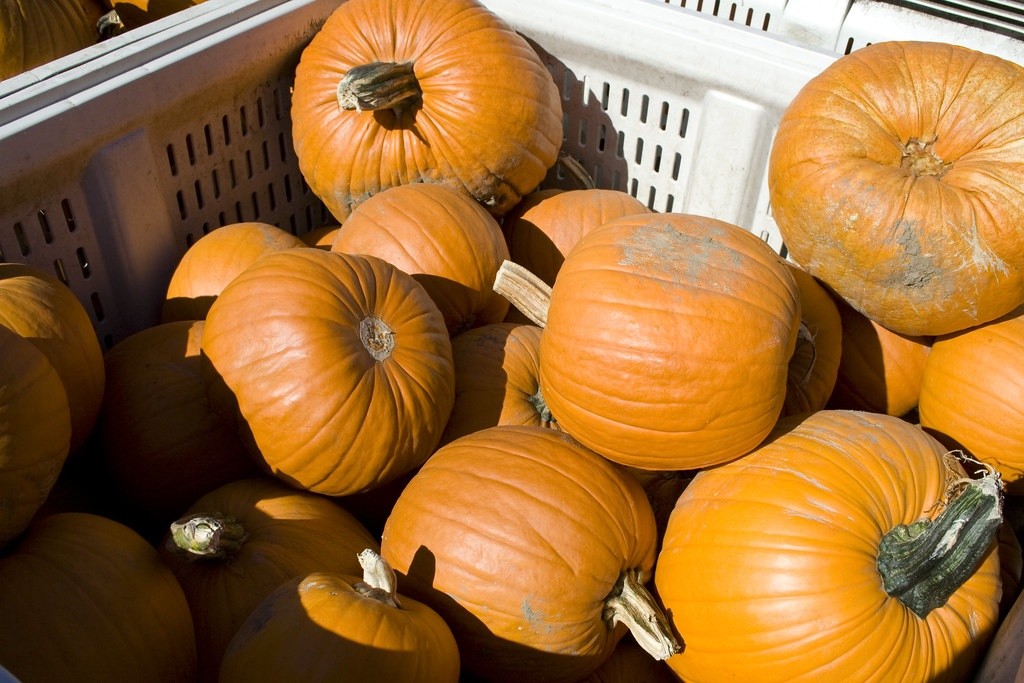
[0,0,1024,683]
